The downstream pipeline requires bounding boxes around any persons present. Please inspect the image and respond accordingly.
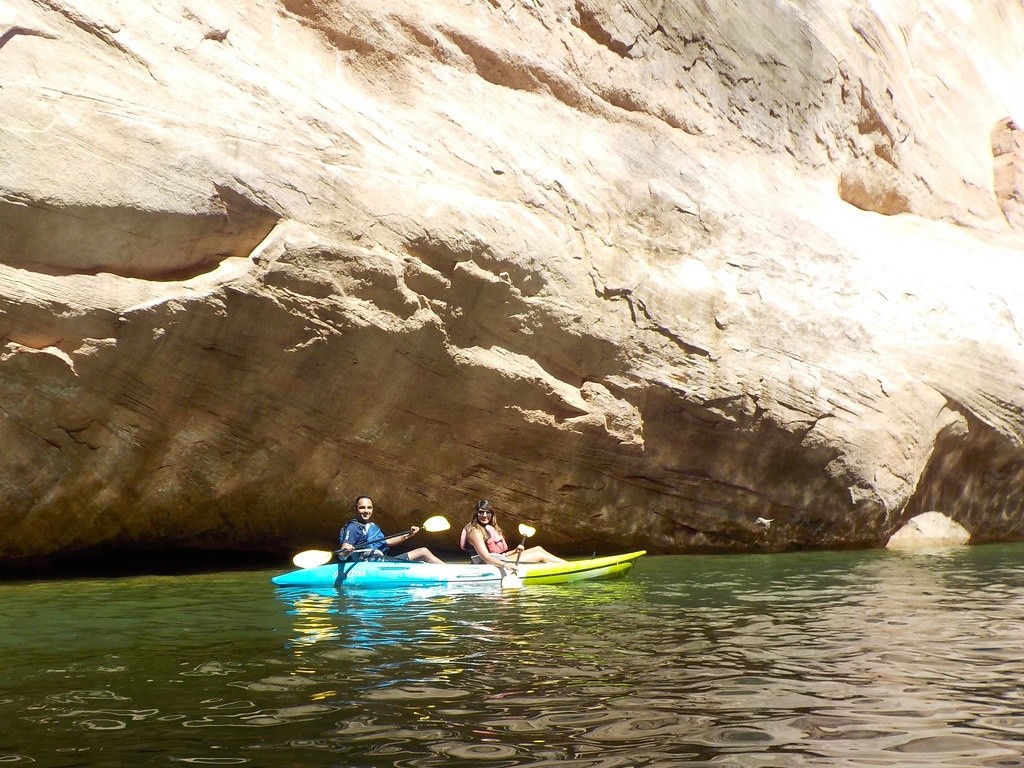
[460,499,596,571]
[338,496,447,564]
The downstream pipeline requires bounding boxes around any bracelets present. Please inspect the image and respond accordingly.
[501,562,505,569]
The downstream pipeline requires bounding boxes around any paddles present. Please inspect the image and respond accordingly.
[293,516,451,568]
[501,523,536,589]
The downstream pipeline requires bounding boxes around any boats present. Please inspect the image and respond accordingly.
[270,550,646,587]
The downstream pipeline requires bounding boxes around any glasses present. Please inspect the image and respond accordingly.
[478,511,492,517]
